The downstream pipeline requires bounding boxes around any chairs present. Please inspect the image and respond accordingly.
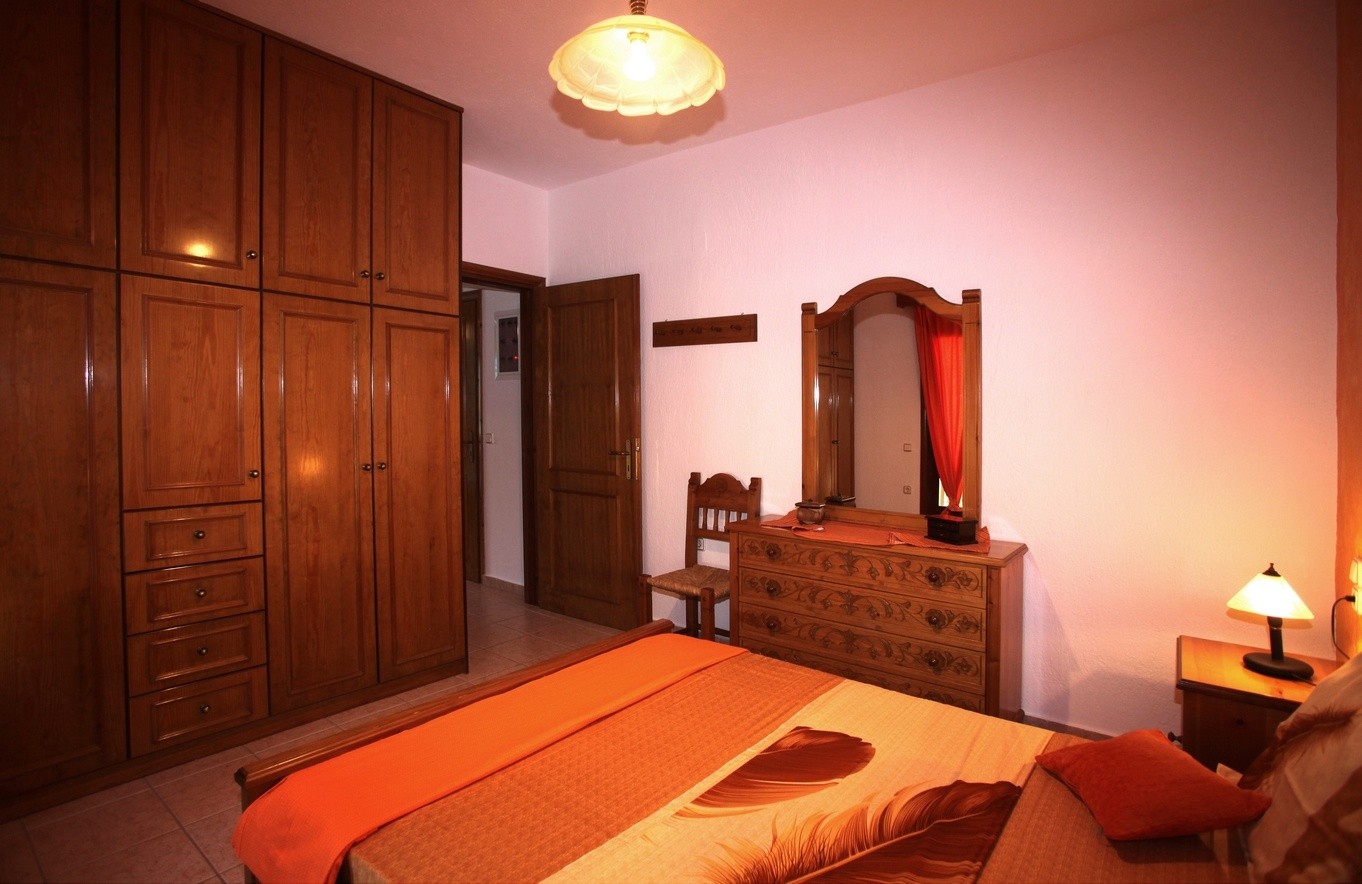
[637,472,763,641]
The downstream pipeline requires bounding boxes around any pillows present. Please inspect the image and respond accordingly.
[1233,652,1362,884]
[1033,724,1272,841]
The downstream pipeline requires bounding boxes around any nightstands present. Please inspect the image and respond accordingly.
[1167,633,1344,772]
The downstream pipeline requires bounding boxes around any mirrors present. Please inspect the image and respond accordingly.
[493,310,522,381]
[800,276,981,535]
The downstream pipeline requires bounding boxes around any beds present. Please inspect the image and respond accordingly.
[232,618,1249,884]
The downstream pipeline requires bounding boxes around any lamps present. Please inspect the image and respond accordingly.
[1225,561,1315,681]
[549,1,726,119]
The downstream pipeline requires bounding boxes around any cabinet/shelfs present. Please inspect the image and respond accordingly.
[118,0,265,289]
[0,251,127,803]
[817,307,857,511]
[725,512,1028,725]
[0,0,119,270]
[261,34,460,317]
[115,271,273,764]
[260,290,467,717]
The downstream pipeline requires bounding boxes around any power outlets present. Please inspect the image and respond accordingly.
[1350,586,1362,616]
[904,485,911,495]
[697,537,705,551]
